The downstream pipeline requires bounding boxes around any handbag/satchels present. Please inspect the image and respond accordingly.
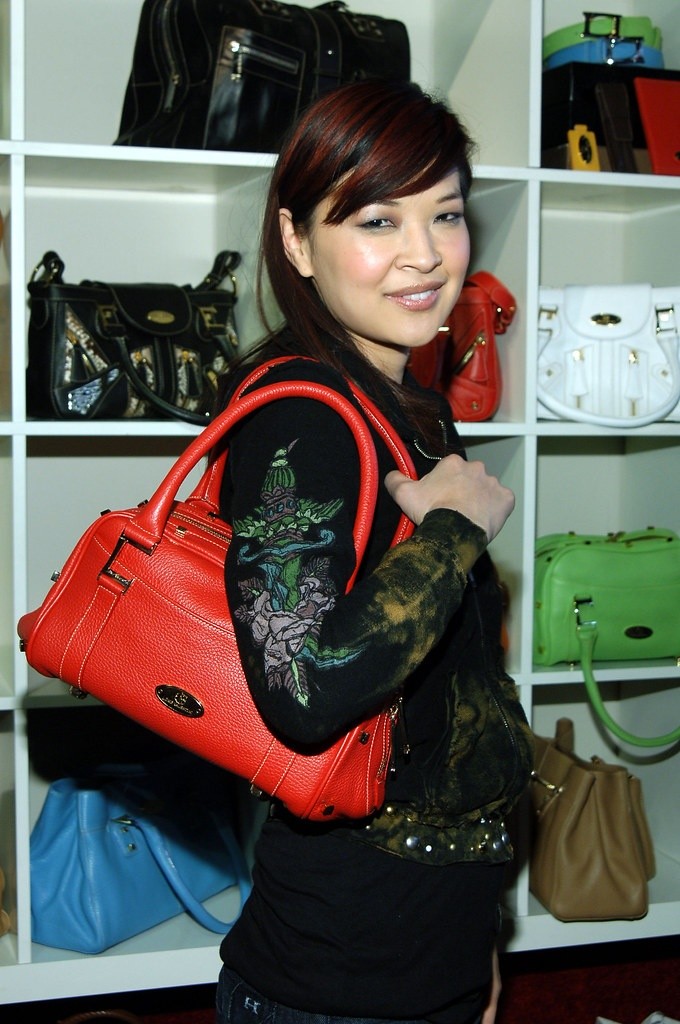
[520,715,658,921]
[26,247,240,428]
[111,0,411,156]
[20,760,254,957]
[19,356,418,824]
[545,11,680,179]
[535,284,679,430]
[534,529,680,747]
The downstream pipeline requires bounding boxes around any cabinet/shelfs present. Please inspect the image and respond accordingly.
[0,0,680,1006]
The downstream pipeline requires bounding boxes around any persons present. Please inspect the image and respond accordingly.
[207,80,542,1024]
